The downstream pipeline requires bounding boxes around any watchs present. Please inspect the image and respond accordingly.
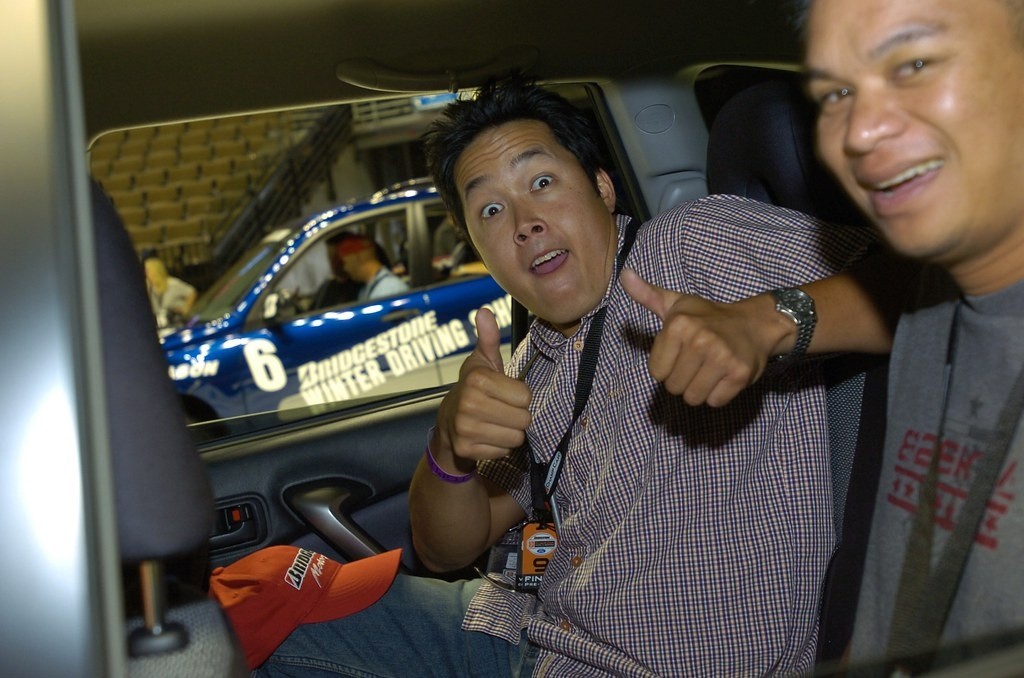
[771,288,816,361]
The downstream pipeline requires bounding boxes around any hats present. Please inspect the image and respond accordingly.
[339,235,376,257]
[211,544,401,668]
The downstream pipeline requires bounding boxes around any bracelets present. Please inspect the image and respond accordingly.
[425,426,478,483]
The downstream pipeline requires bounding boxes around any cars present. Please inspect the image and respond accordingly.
[159,176,514,437]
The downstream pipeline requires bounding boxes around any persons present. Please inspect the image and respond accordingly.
[142,254,197,328]
[209,77,916,678]
[790,0,1024,678]
[306,231,411,314]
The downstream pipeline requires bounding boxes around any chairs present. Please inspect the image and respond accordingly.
[706,83,845,225]
[88,178,213,657]
